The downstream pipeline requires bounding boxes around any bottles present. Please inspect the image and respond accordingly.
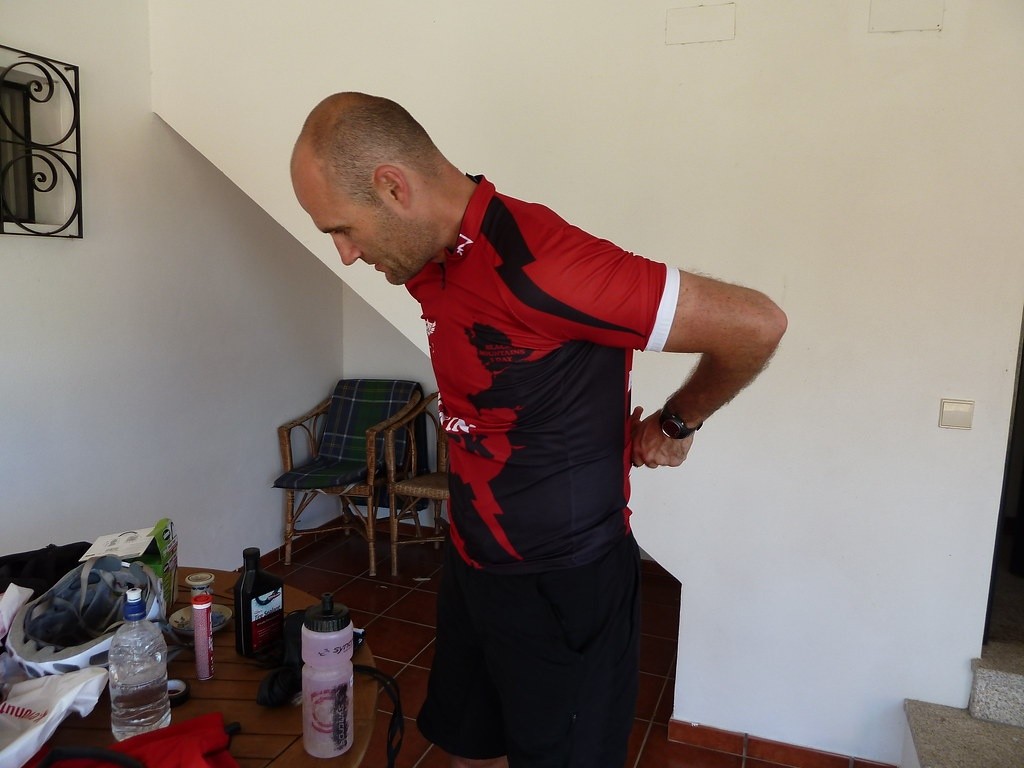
[109,588,172,742]
[301,592,355,760]
[233,547,287,656]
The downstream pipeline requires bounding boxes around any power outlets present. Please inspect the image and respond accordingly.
[939,399,976,430]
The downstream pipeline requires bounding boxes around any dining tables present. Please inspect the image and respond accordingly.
[47,564,380,768]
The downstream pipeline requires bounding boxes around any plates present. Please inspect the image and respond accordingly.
[169,604,234,637]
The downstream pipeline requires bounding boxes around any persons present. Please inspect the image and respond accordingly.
[290,92,790,767]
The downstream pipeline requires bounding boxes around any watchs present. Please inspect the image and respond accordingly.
[658,402,704,439]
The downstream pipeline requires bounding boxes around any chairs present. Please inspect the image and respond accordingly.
[270,377,430,577]
[384,392,451,577]
